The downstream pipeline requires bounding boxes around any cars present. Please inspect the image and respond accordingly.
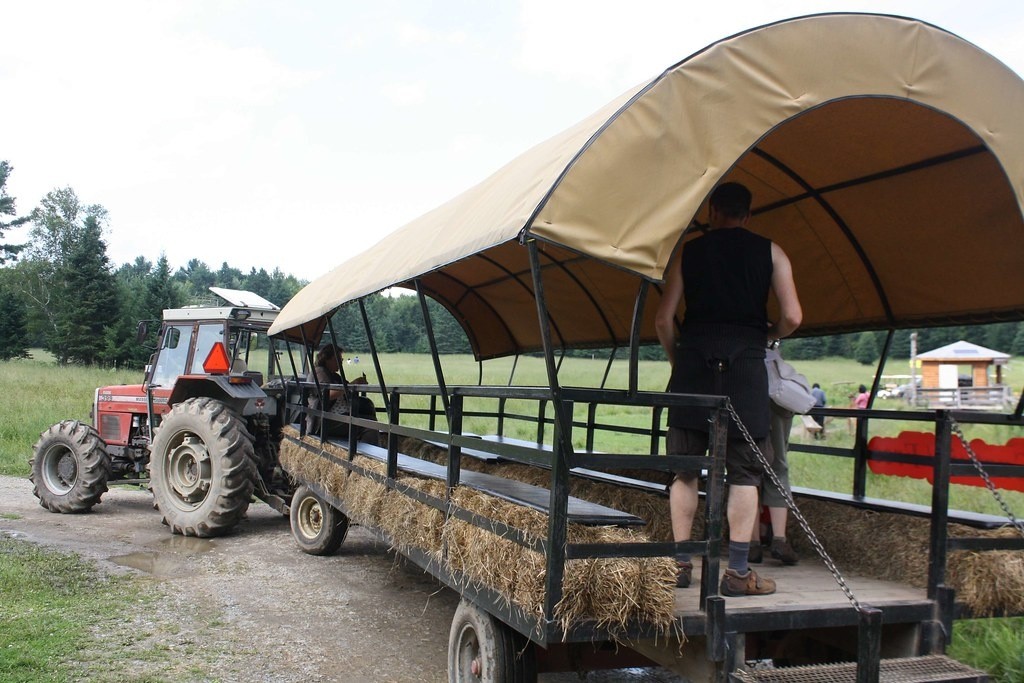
[878,375,974,401]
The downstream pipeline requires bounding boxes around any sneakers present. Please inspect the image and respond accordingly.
[720,565,776,596]
[676,562,691,587]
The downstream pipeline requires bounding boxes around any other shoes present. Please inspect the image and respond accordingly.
[748,545,762,562]
[772,539,799,563]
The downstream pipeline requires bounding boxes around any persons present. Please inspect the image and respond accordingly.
[304,342,380,446]
[347,358,352,364]
[809,381,829,442]
[847,385,872,436]
[747,343,800,566]
[353,356,360,364]
[651,182,805,597]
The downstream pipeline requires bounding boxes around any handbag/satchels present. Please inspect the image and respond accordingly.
[764,349,817,414]
[329,395,348,415]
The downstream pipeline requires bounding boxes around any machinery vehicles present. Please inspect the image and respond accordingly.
[28,285,378,537]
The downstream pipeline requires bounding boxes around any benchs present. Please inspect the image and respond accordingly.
[432,429,1024,531]
[289,422,648,525]
[885,384,899,392]
